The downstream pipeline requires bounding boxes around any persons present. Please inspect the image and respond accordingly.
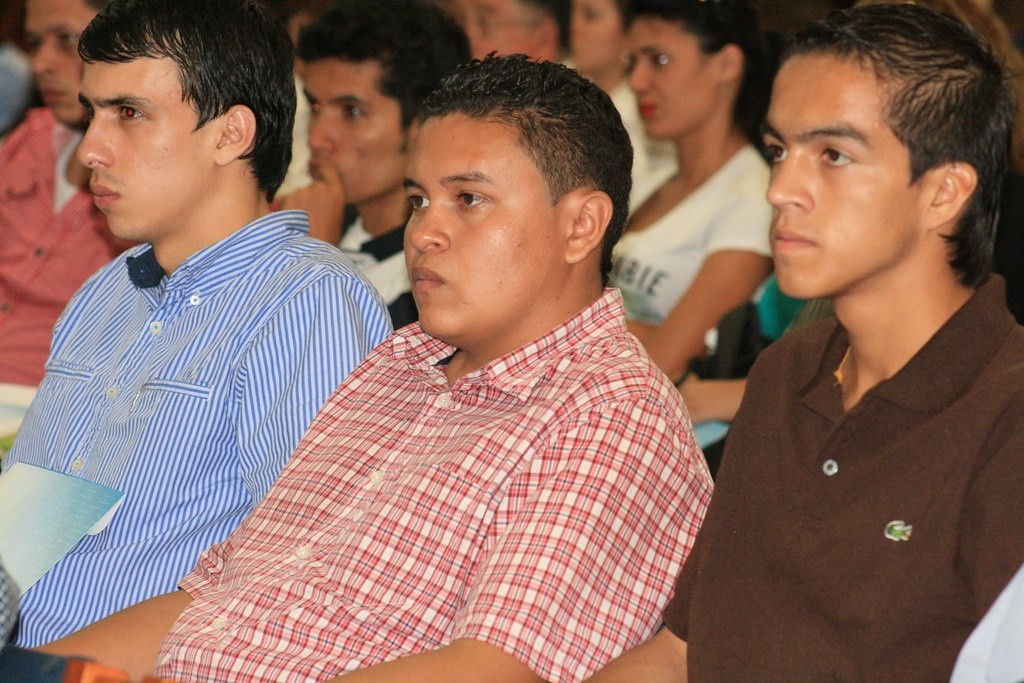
[0,0,143,390]
[43,52,714,683]
[279,0,472,332]
[461,0,776,385]
[0,0,394,648]
[585,0,1024,683]
